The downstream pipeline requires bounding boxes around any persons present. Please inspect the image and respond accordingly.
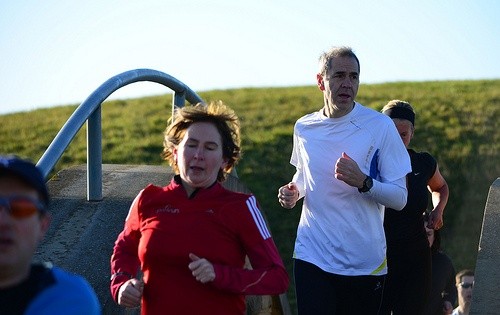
[276,49,413,315]
[0,154,104,315]
[451,269,475,315]
[109,101,290,315]
[379,101,449,315]
[422,210,456,315]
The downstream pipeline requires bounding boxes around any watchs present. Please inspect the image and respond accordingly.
[358,176,374,193]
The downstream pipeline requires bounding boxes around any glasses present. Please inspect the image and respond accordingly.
[457,282,472,289]
[0,195,46,218]
